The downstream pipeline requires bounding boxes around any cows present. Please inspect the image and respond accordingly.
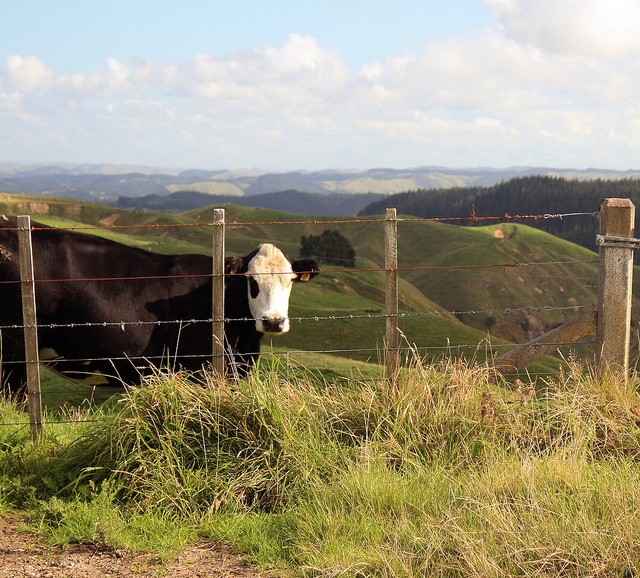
[0,213,320,412]
